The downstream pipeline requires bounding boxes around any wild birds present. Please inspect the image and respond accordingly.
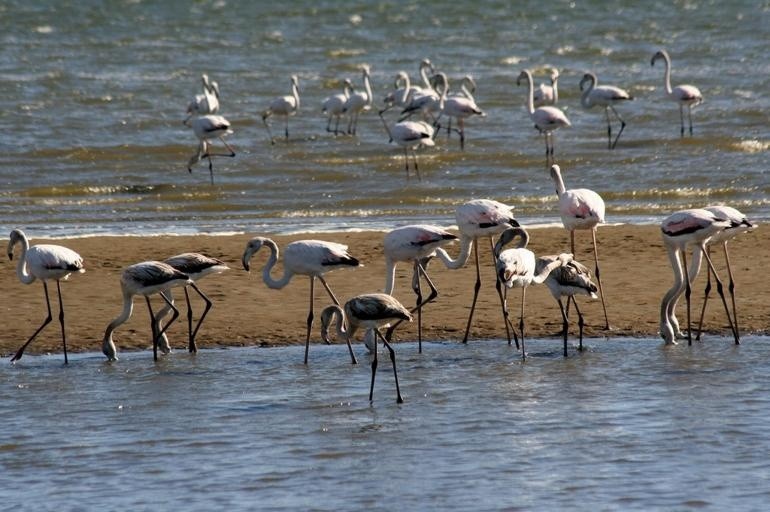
[578,71,634,151]
[495,227,598,359]
[6,227,88,365]
[242,236,365,366]
[497,246,575,361]
[516,69,571,168]
[319,56,486,183]
[183,73,238,175]
[667,204,754,342]
[320,292,414,405]
[532,66,561,109]
[650,50,703,138]
[381,223,457,359]
[548,165,611,330]
[101,260,214,362]
[659,208,741,346]
[151,252,232,356]
[261,73,302,145]
[432,198,514,349]
